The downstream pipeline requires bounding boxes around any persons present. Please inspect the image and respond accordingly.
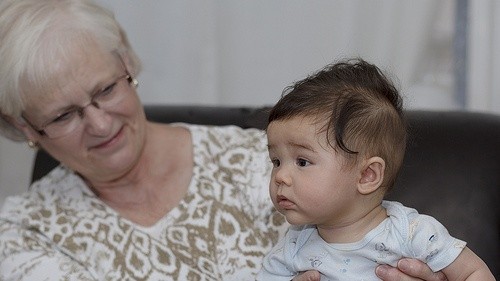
[0,0,446,281]
[254,58,496,281]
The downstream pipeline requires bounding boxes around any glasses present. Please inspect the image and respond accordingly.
[25,68,139,139]
